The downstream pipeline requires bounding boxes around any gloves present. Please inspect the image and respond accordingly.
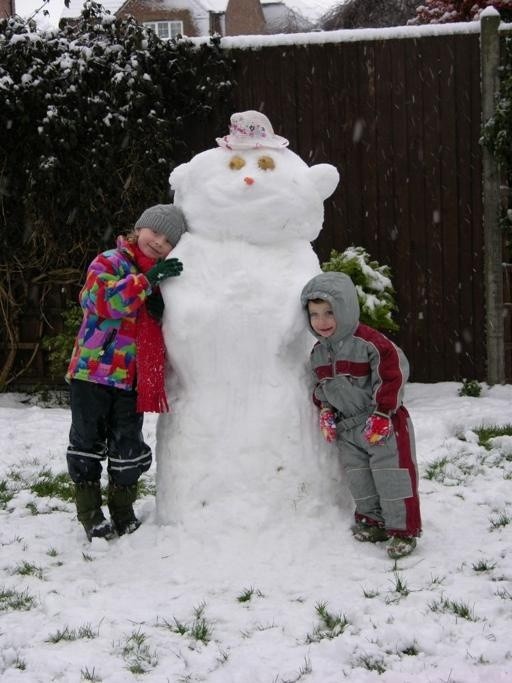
[364,412,391,447]
[319,407,338,445]
[143,257,184,287]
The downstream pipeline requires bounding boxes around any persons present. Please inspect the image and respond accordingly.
[299,271,422,558]
[62,203,186,543]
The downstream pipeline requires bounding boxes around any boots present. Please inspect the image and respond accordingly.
[72,481,116,542]
[107,476,142,535]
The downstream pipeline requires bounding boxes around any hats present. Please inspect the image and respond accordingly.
[135,203,187,247]
[215,109,289,151]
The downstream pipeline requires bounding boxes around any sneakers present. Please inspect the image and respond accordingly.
[388,536,416,558]
[355,524,392,541]
